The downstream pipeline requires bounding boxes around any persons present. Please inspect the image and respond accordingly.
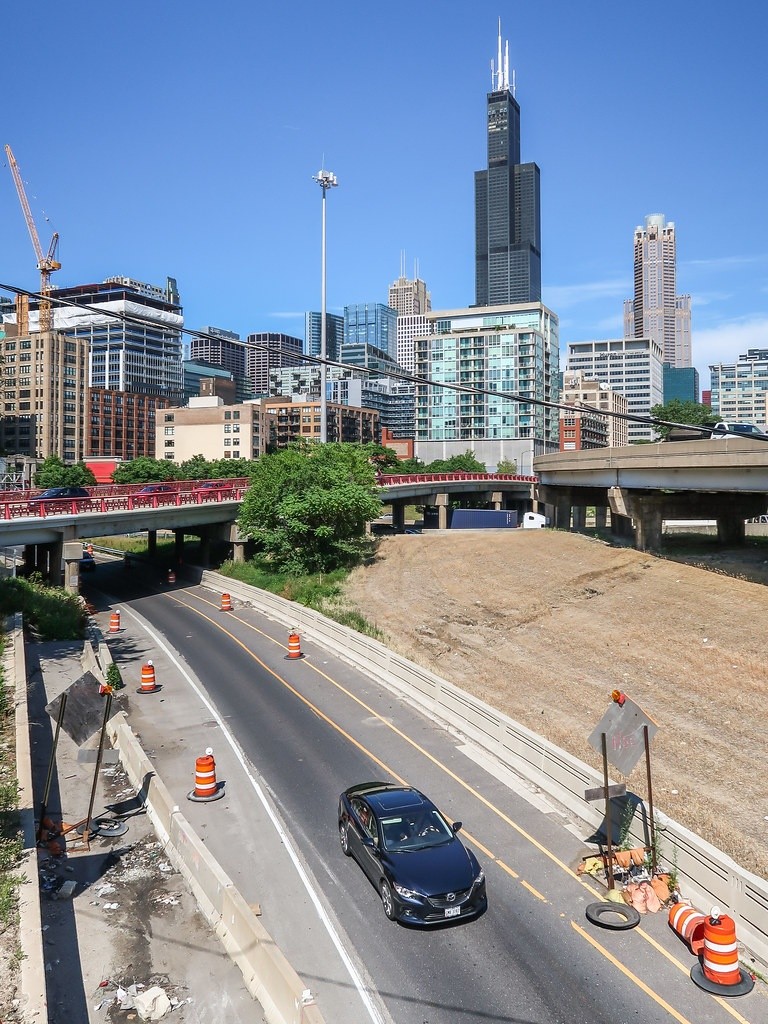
[409,815,432,836]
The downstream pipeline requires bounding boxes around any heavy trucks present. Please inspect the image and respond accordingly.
[423,509,546,528]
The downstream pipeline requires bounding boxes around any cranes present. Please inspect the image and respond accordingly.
[3,144,62,332]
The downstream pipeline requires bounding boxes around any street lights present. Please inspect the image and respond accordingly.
[513,458,517,466]
[521,449,535,477]
[312,150,338,451]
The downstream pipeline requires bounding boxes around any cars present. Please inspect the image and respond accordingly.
[133,485,181,504]
[338,782,487,928]
[191,482,229,496]
[371,524,424,534]
[28,486,91,511]
[77,550,96,572]
[379,513,393,519]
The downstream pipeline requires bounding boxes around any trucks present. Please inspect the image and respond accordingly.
[708,420,766,439]
